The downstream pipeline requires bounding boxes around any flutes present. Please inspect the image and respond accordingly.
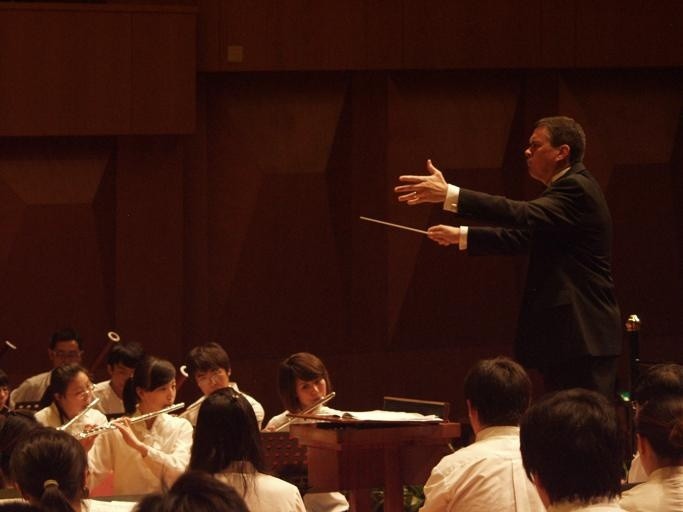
[56,397,99,431]
[71,402,185,441]
[269,392,337,433]
[178,385,233,419]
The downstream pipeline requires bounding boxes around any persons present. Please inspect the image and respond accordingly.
[1,328,351,511]
[619,396,682,512]
[393,115,623,399]
[624,360,682,486]
[417,356,548,511]
[519,386,633,511]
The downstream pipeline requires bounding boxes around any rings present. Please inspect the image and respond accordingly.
[413,190,417,198]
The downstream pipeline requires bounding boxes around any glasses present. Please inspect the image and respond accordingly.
[197,371,227,387]
[66,383,97,401]
